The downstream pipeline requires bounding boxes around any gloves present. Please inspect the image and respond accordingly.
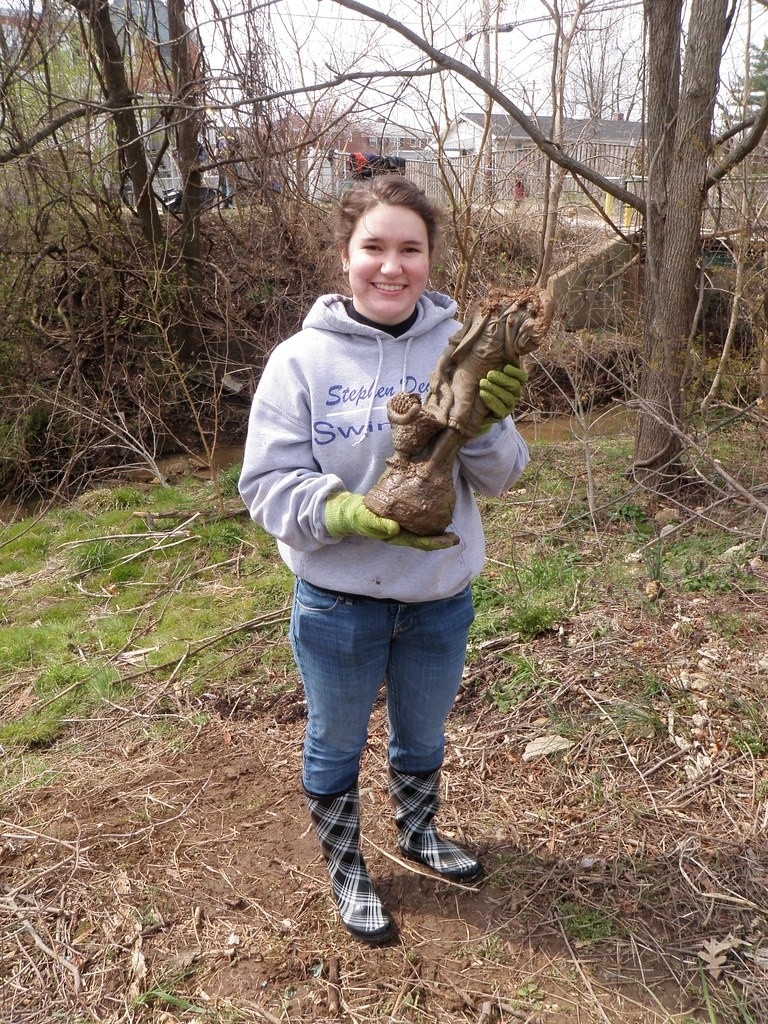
[471,364,529,436]
[324,491,460,551]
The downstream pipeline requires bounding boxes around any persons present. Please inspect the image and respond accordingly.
[239,176,531,943]
[417,290,557,490]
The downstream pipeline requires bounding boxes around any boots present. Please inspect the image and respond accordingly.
[388,764,483,883]
[301,774,397,944]
